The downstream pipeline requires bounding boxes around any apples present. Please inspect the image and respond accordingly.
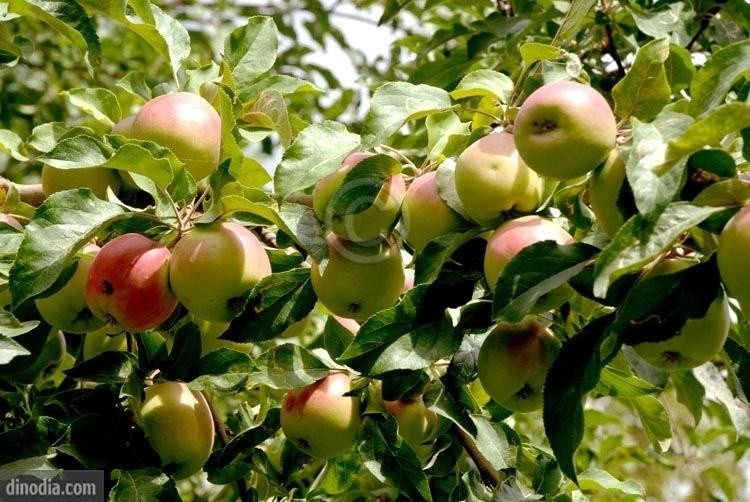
[0,212,24,231]
[137,381,215,481]
[282,371,362,460]
[476,319,563,414]
[382,397,437,442]
[40,158,120,204]
[317,81,614,320]
[628,202,750,372]
[30,220,272,331]
[108,91,224,185]
